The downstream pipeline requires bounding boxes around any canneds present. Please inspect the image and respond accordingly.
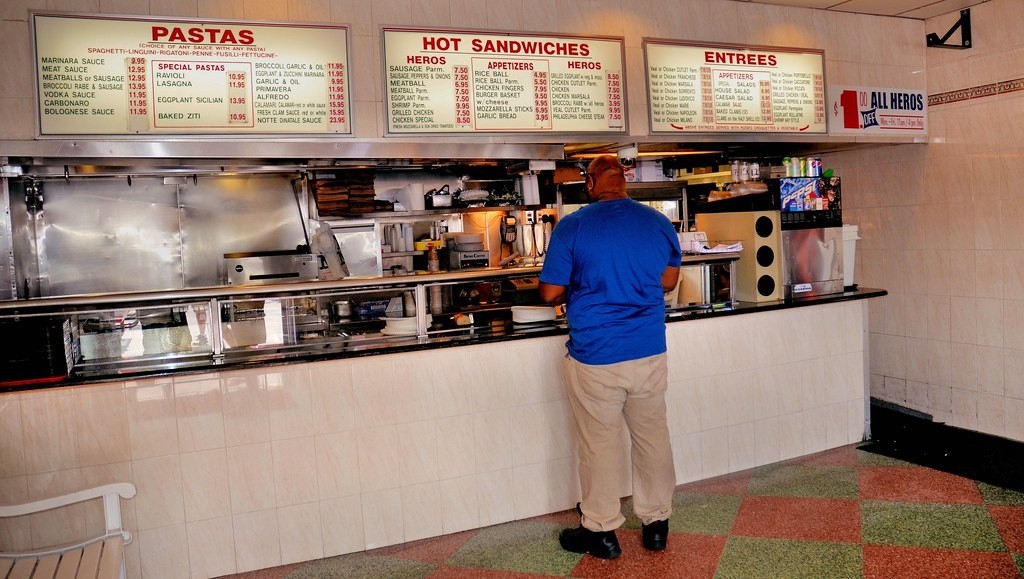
[782,157,823,178]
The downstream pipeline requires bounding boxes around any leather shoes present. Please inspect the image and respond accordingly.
[642,518,668,550]
[559,524,621,559]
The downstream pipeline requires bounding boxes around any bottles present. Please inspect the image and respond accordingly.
[498,251,520,265]
[384,223,414,252]
[404,291,415,316]
[748,163,760,178]
[739,162,748,180]
[731,160,739,182]
[428,246,439,271]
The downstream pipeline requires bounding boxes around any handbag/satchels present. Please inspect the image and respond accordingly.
[291,178,350,280]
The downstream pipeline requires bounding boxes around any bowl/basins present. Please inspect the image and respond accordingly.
[453,243,484,251]
[454,233,484,243]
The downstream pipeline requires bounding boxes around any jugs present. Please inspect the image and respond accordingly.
[330,299,356,316]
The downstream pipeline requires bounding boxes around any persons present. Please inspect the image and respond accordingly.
[538,155,682,559]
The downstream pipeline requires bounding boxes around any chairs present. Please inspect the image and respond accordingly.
[0,482,134,579]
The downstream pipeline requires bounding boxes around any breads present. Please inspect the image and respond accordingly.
[316,179,392,214]
[454,313,470,325]
